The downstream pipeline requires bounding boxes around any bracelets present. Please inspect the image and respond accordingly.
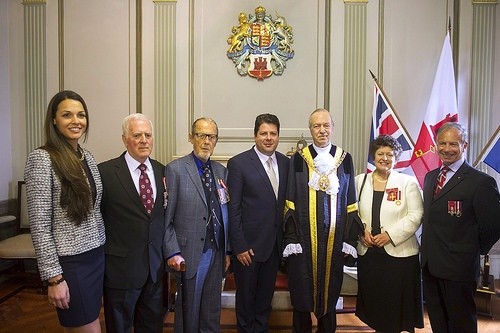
[47,277,64,286]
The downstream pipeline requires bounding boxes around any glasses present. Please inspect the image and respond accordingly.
[194,133,217,140]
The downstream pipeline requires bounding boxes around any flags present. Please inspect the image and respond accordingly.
[409,31,460,193]
[366,84,414,176]
[481,128,500,175]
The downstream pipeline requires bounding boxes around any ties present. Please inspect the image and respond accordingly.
[267,156,278,201]
[201,165,219,250]
[138,164,155,217]
[433,167,450,199]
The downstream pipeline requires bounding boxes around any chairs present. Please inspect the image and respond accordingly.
[0,181,48,294]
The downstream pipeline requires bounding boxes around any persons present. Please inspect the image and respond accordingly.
[283,108,360,333]
[227,113,290,333]
[421,122,500,333]
[355,134,425,333]
[97,113,168,333]
[164,117,230,333]
[23,90,106,333]
